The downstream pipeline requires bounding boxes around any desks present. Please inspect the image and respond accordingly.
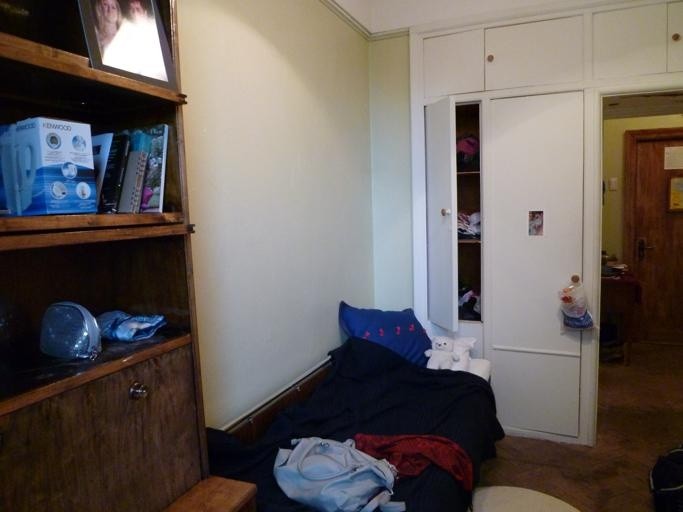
[601,273,641,367]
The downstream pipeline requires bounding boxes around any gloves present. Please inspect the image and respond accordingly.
[95,310,165,341]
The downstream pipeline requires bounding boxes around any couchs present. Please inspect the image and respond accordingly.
[221,337,501,512]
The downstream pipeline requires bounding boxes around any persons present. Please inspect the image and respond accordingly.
[95,0,124,59]
[128,1,149,22]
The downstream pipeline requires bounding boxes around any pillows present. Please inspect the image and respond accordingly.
[339,301,432,364]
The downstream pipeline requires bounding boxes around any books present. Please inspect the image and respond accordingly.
[88,123,169,213]
[605,260,630,275]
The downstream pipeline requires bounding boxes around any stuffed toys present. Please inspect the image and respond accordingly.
[425,336,460,371]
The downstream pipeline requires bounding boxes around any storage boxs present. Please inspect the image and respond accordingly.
[6,116,96,216]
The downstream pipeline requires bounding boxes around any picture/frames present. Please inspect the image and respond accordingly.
[74,0,175,85]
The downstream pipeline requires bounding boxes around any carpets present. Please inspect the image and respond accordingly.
[468,484,587,511]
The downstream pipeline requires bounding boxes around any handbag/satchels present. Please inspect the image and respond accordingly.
[273,437,406,512]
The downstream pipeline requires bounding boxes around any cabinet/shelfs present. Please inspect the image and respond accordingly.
[418,13,589,95]
[587,1,681,93]
[0,1,214,512]
[413,93,602,449]
